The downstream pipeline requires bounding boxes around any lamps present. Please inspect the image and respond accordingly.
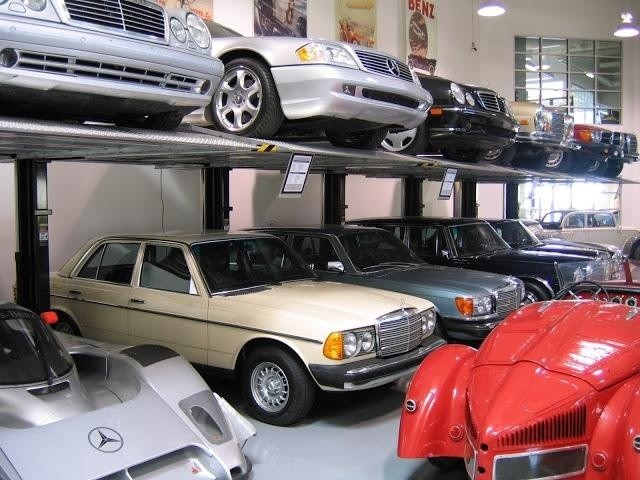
[612,9,640,38]
[476,1,508,17]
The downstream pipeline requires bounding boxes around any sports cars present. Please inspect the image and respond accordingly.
[397,252,640,480]
[0,300,259,480]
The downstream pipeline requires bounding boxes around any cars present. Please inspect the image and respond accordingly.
[0,0,224,131]
[228,223,525,349]
[479,100,582,170]
[13,230,448,428]
[378,71,520,163]
[344,209,640,306]
[181,18,434,151]
[542,123,640,177]
